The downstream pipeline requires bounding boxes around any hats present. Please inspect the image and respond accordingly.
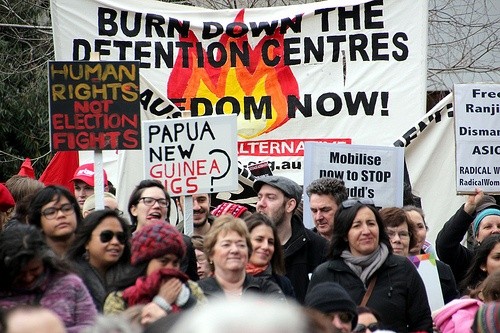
[253,175,303,203]
[472,208,500,238]
[305,282,358,331]
[210,202,249,219]
[475,195,500,212]
[130,220,186,268]
[67,163,108,187]
[0,183,16,212]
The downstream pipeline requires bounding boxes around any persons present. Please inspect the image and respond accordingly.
[403,204,427,258]
[306,178,348,245]
[102,218,208,318]
[0,164,500,333]
[252,175,330,306]
[27,185,84,258]
[191,213,288,307]
[379,207,445,312]
[67,208,134,291]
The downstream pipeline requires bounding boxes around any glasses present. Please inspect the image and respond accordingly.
[322,313,355,323]
[384,229,413,238]
[40,203,76,220]
[196,256,208,263]
[99,230,127,244]
[338,198,375,209]
[353,322,380,333]
[88,205,123,217]
[138,197,170,208]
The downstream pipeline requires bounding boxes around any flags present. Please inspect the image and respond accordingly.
[116,71,184,228]
[391,93,469,256]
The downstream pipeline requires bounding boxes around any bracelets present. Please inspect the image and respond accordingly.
[152,296,172,314]
